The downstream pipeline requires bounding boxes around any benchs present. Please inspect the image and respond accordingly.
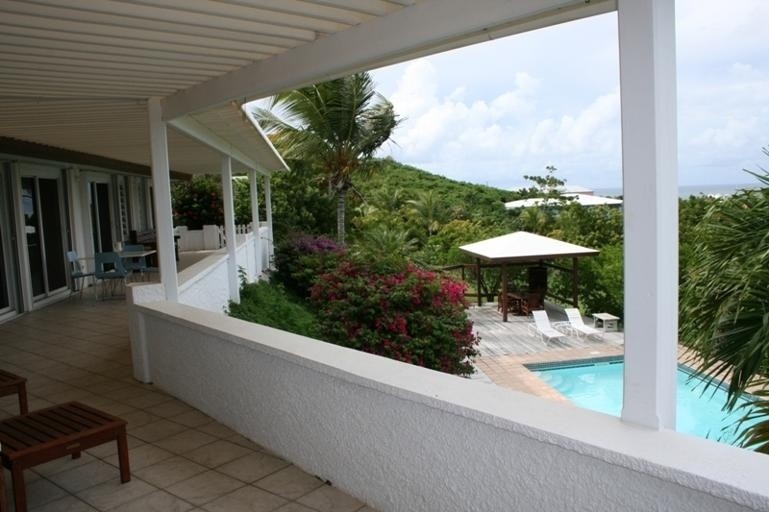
[129,228,182,270]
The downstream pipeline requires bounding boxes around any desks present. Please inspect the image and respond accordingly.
[591,312,620,333]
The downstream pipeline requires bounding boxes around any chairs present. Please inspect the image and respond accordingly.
[65,243,166,301]
[559,306,605,343]
[497,286,545,319]
[526,308,572,349]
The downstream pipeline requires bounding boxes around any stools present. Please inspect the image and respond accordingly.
[1,400,132,512]
[0,367,31,414]
